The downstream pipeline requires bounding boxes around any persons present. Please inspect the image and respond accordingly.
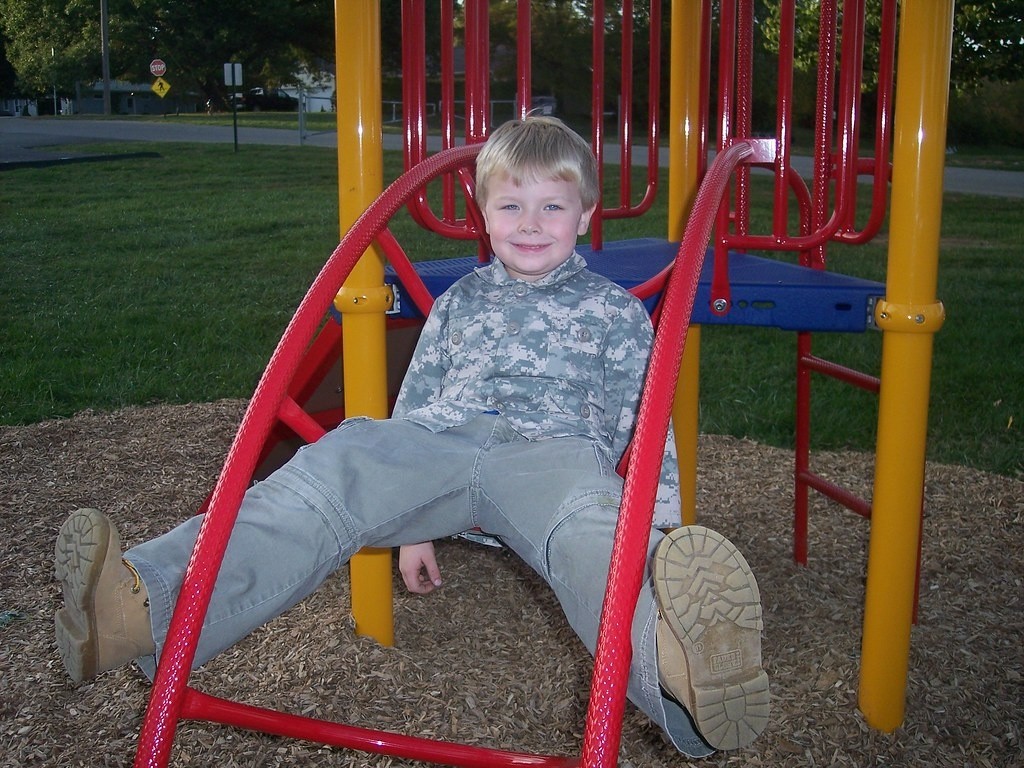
[56,116,772,759]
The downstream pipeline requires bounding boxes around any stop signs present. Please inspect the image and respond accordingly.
[149,59,167,77]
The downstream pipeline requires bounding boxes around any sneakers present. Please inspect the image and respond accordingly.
[654,525,771,751]
[53,508,155,684]
